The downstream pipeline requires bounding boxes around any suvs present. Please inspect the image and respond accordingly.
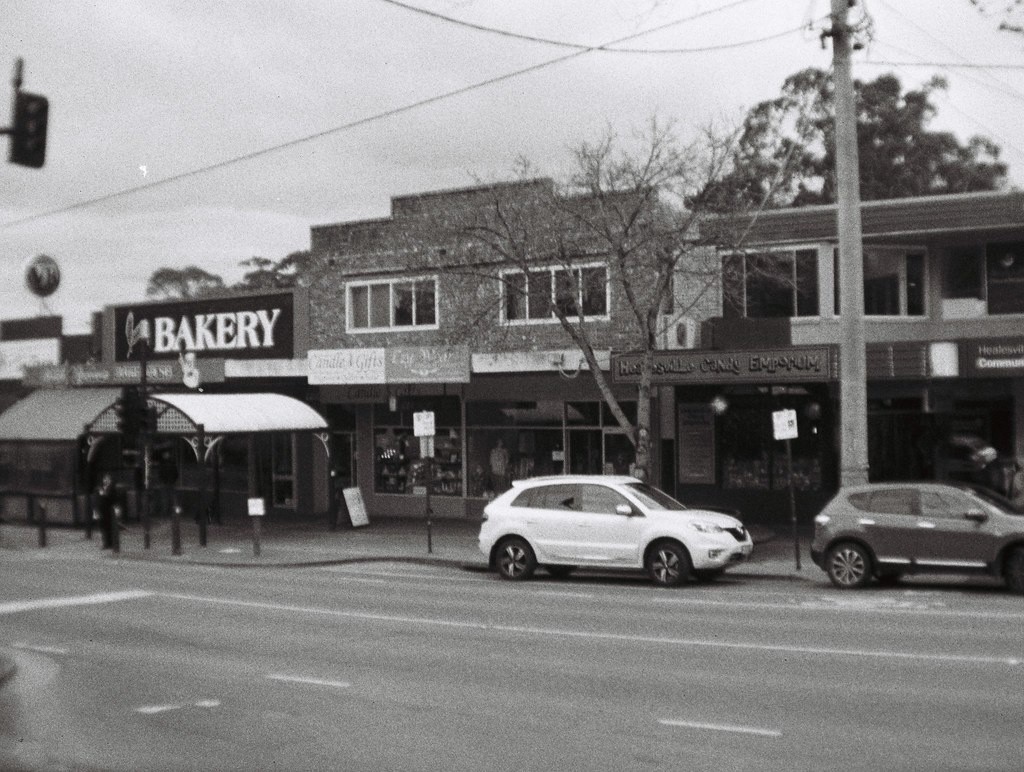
[478,474,754,589]
[810,482,1024,599]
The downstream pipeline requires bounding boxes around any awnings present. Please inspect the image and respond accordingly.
[0,386,122,439]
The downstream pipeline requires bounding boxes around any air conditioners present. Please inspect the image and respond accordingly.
[656,314,696,349]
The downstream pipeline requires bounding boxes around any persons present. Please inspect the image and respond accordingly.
[91,473,123,554]
[469,463,490,496]
[486,438,509,495]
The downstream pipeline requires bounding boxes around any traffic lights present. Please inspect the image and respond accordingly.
[8,93,49,168]
[141,408,153,437]
[115,390,131,431]
